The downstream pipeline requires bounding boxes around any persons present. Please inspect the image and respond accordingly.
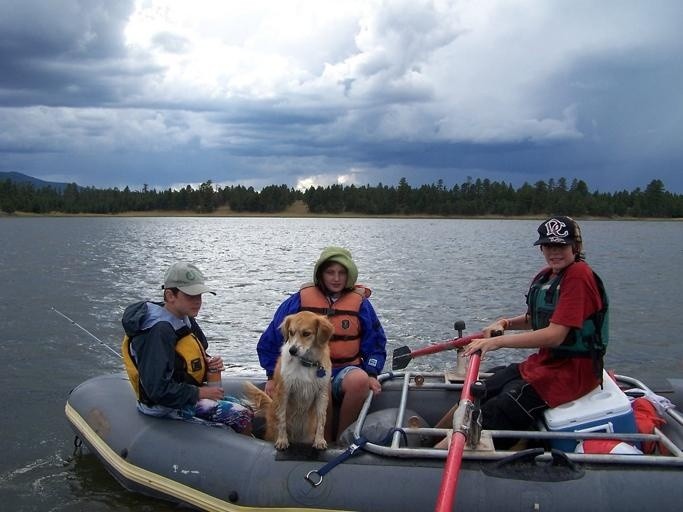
[257,246,386,453]
[431,215,608,452]
[122,260,254,439]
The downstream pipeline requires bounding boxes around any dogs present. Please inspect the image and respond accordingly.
[245,309,336,451]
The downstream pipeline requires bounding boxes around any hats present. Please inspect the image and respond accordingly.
[534,217,582,245]
[162,263,216,296]
[314,247,358,288]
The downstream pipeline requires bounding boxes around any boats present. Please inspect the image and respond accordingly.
[63,365,682,512]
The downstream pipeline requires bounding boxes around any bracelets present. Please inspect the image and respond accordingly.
[501,319,511,329]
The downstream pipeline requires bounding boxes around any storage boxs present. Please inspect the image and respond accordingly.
[543,368,644,453]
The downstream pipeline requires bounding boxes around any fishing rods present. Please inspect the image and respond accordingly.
[49,305,124,362]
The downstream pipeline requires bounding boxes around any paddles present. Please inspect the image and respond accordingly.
[392,329,505,370]
